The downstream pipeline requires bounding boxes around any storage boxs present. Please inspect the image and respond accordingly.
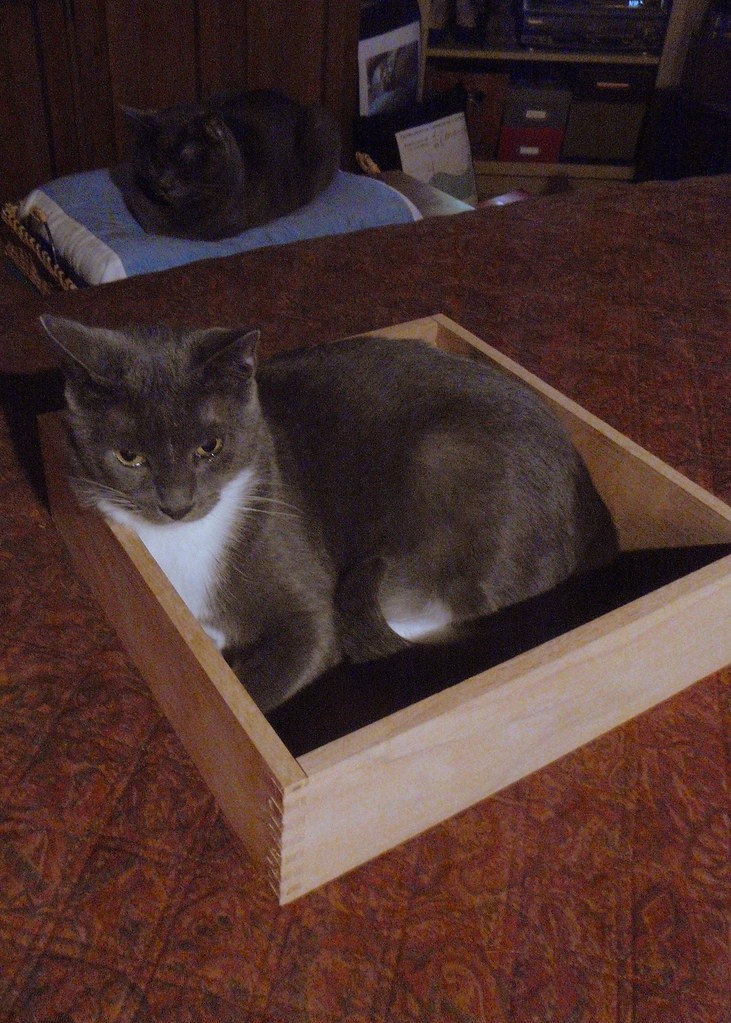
[563,98,645,162]
[497,123,565,165]
[502,86,572,127]
[36,311,731,907]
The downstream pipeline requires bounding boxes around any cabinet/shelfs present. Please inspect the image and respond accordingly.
[414,0,702,202]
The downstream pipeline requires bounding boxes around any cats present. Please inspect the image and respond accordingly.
[38,312,627,719]
[119,87,327,242]
